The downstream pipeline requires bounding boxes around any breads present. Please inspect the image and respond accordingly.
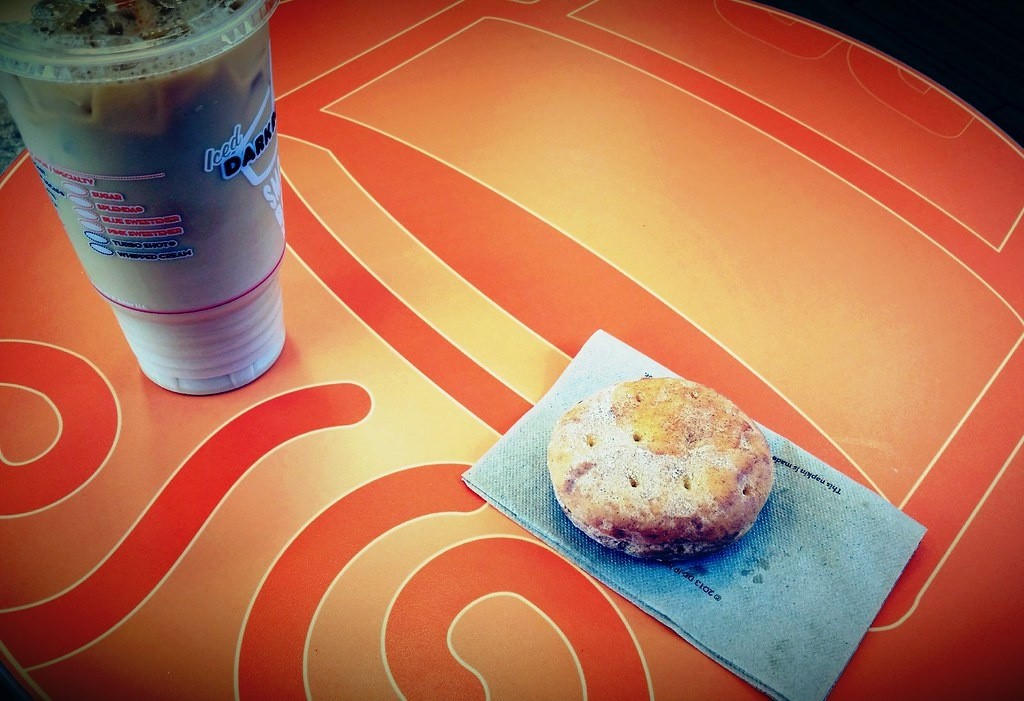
[546,377,775,559]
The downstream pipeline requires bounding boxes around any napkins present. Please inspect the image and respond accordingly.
[459,328,929,701]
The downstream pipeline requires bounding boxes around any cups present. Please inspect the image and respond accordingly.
[1,1,286,395]
